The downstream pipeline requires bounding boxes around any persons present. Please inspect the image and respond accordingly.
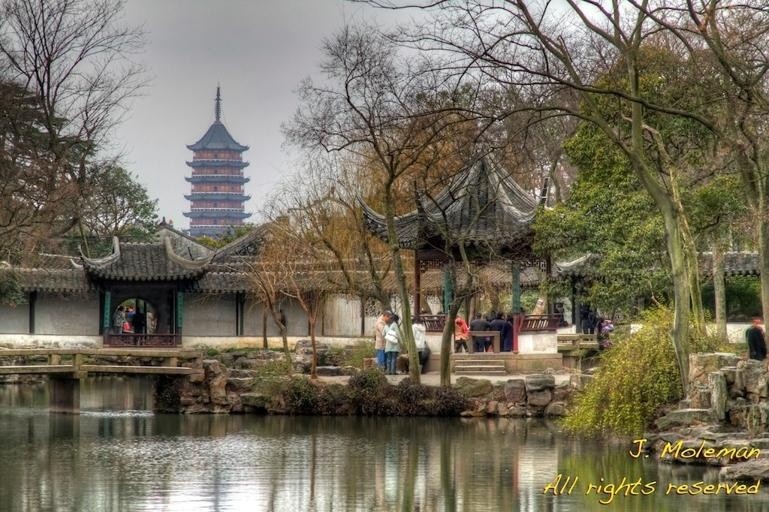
[454,310,524,353]
[375,310,430,376]
[113,304,156,344]
[745,314,765,361]
[556,299,613,348]
[276,307,287,335]
